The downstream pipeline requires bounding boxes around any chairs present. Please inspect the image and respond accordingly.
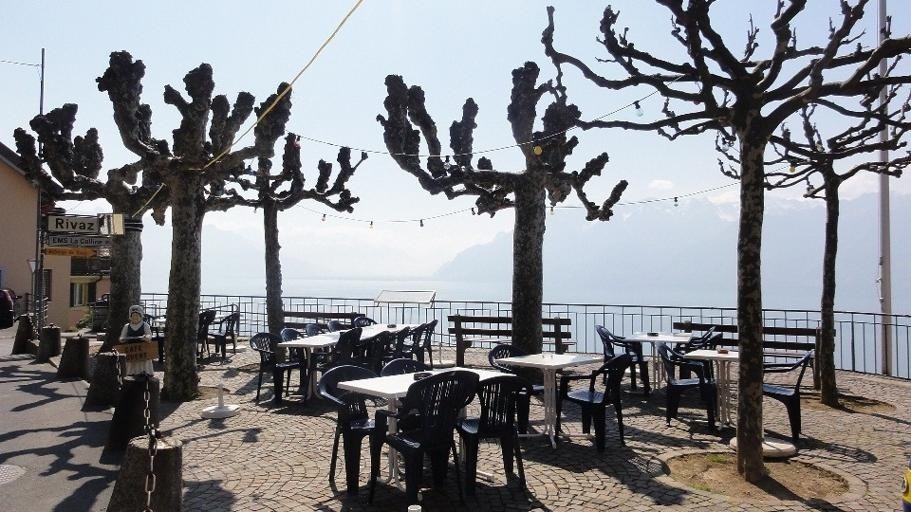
[368,369,481,506]
[674,332,724,380]
[555,352,635,458]
[593,324,649,389]
[667,325,717,342]
[655,341,718,430]
[762,349,817,447]
[489,345,550,433]
[318,365,392,502]
[453,374,534,498]
[248,316,439,402]
[143,310,241,361]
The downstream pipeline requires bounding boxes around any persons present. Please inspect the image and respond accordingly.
[119,305,154,377]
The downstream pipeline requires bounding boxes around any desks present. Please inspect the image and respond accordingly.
[495,351,606,450]
[621,329,696,388]
[683,349,739,435]
[338,363,516,494]
